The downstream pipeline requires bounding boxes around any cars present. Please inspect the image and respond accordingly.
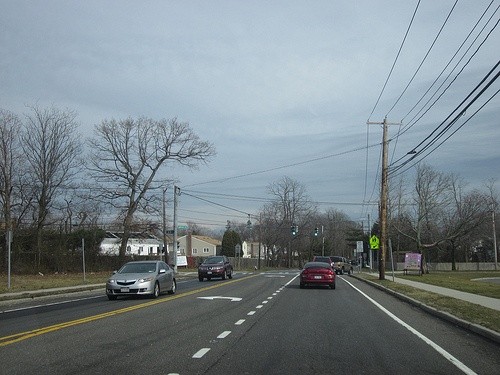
[299,262,336,289]
[106,260,177,300]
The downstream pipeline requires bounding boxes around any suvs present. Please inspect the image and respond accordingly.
[330,255,354,275]
[198,254,233,282]
[312,256,334,271]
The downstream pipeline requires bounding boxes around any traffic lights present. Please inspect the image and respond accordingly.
[314,227,319,235]
[292,225,295,236]
